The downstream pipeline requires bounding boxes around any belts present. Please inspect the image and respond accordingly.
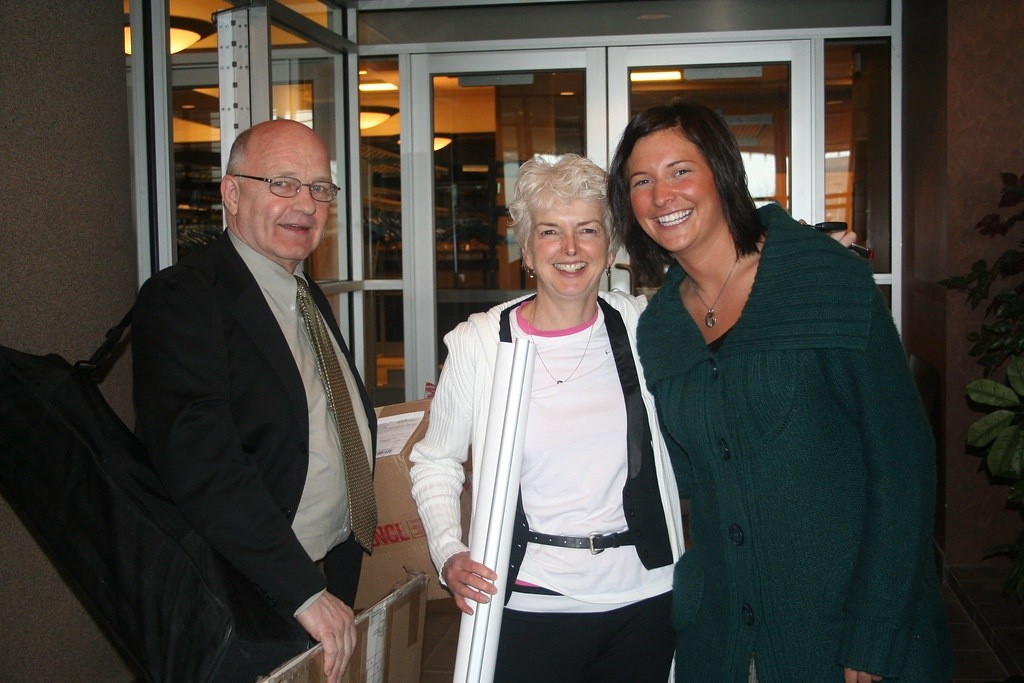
[528,530,633,555]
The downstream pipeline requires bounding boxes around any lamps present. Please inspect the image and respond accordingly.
[397,135,453,152]
[124,12,217,56]
[359,106,399,130]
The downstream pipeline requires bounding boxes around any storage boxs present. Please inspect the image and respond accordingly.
[353,396,472,612]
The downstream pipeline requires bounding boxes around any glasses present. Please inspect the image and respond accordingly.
[231,173,341,203]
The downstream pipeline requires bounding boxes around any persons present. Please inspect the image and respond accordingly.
[408,154,688,683]
[134,117,377,683]
[607,99,945,683]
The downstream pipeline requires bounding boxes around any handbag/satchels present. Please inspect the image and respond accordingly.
[0,260,315,682]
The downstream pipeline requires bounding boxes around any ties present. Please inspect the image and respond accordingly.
[293,273,379,556]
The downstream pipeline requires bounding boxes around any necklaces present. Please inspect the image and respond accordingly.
[689,258,738,328]
